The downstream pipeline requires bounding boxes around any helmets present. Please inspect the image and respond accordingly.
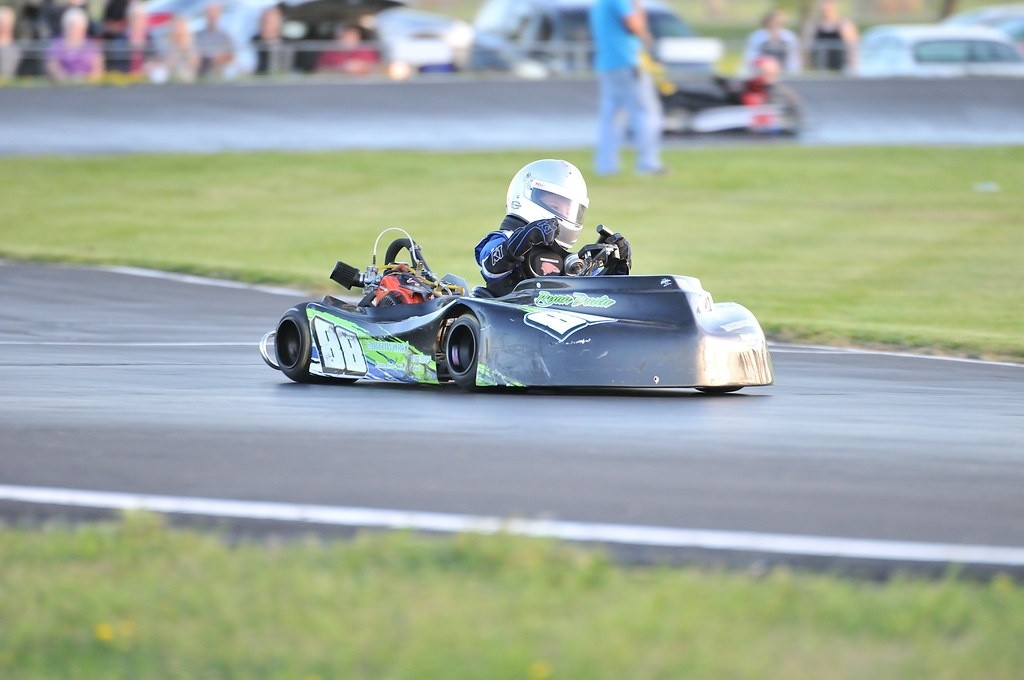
[506,158,589,249]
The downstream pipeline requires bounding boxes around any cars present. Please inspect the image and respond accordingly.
[835,22,1024,78]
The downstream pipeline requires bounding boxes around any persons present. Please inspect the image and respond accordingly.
[474,159,632,299]
[737,0,860,73]
[589,0,673,179]
[721,54,779,106]
[0,0,411,83]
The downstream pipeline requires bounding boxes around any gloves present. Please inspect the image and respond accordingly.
[604,232,631,273]
[506,217,560,258]
